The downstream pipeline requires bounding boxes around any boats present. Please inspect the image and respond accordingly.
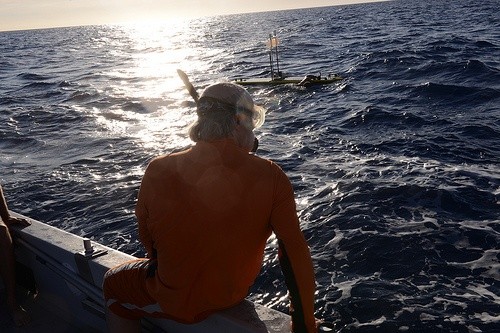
[232,31,343,90]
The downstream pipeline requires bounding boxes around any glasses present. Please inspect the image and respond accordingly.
[251,106,265,130]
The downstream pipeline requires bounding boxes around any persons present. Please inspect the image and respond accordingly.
[0,184,30,329]
[102,82,321,333]
[298,73,337,86]
